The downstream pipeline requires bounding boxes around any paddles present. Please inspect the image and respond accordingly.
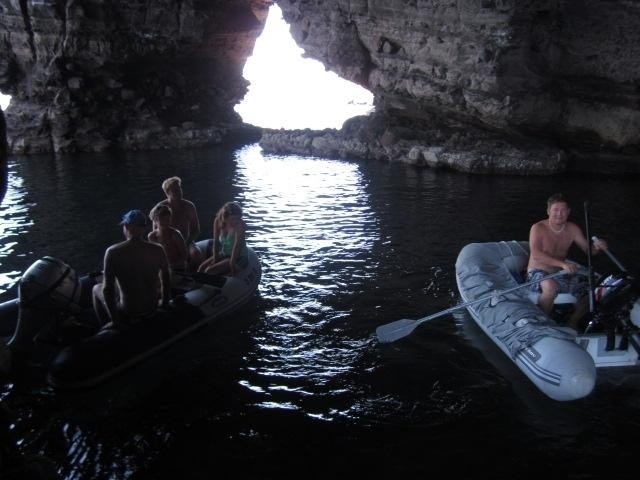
[376,270,567,343]
[171,271,226,288]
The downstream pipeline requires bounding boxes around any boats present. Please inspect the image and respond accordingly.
[0,237,263,398]
[453,239,639,405]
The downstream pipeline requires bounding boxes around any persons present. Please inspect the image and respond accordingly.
[91,209,174,330]
[523,193,609,329]
[148,176,203,271]
[148,205,190,299]
[197,201,247,277]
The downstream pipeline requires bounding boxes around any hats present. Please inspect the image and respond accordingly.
[149,204,172,220]
[117,208,149,226]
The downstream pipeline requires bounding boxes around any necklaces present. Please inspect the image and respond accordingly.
[549,220,567,233]
